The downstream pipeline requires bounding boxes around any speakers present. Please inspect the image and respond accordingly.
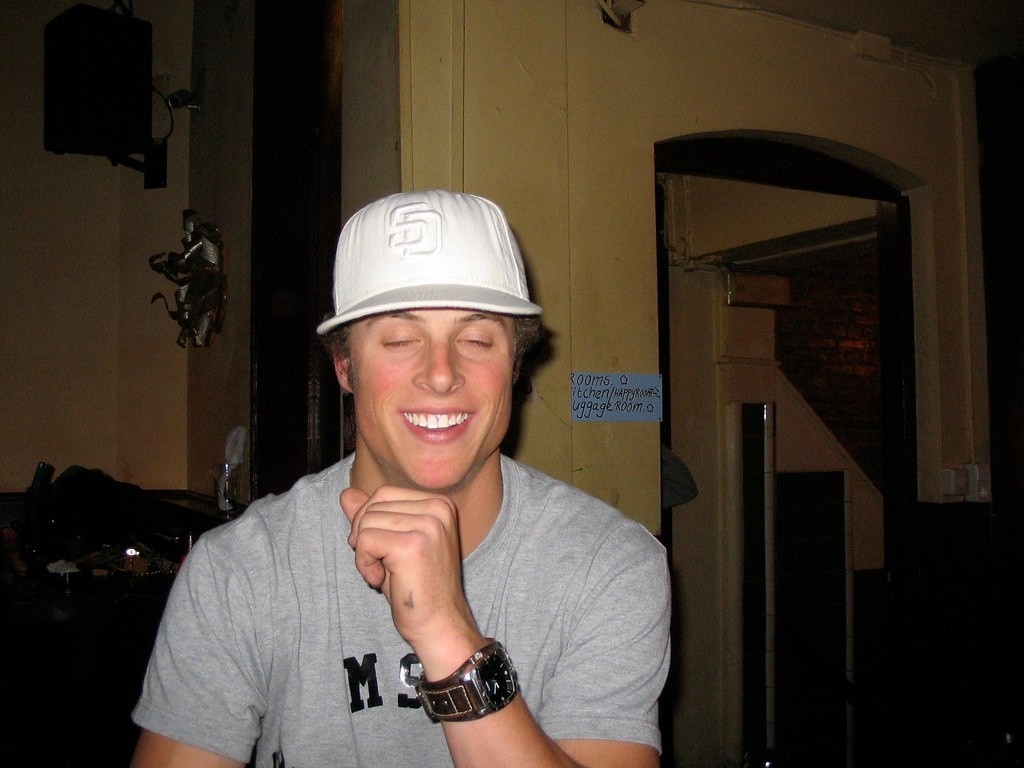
[44,3,155,155]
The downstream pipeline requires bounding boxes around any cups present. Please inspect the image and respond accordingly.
[213,463,242,514]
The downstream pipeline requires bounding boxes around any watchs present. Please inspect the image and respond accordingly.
[415,637,519,725]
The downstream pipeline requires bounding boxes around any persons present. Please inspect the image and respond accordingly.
[131,189,673,768]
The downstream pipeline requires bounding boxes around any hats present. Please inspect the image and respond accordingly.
[317,190,544,336]
